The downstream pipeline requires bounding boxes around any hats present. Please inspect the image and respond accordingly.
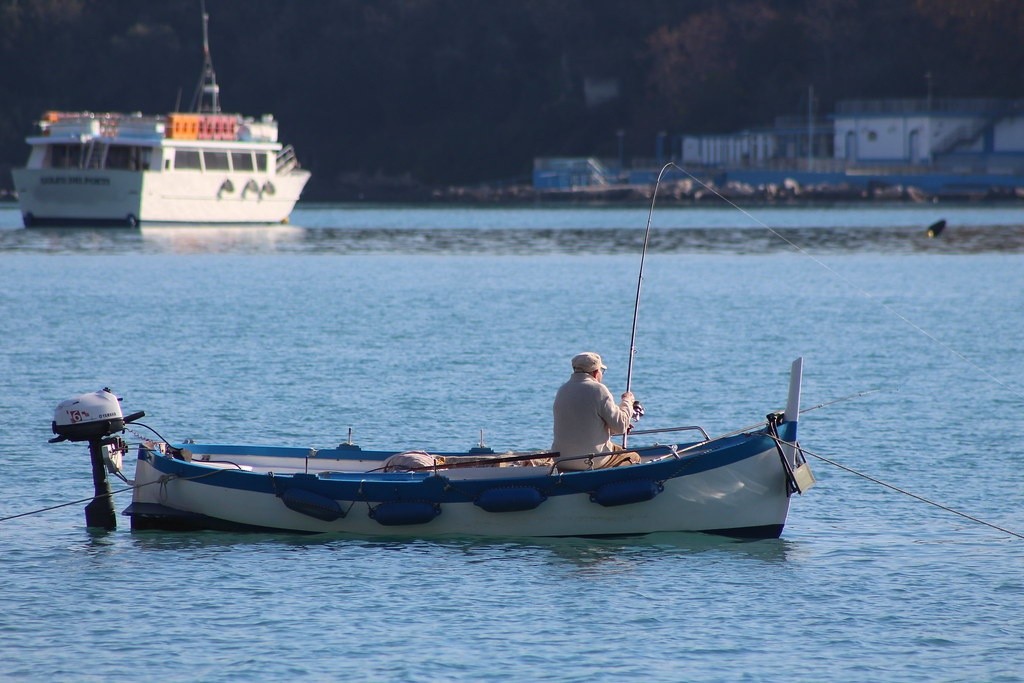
[571,352,607,373]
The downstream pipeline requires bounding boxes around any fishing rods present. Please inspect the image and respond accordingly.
[622,157,723,428]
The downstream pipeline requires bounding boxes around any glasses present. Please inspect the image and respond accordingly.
[600,368,606,374]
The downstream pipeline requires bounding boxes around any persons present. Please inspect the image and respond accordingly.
[551,352,640,471]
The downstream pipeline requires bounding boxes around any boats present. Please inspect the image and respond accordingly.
[9,2,311,227]
[50,355,807,542]
